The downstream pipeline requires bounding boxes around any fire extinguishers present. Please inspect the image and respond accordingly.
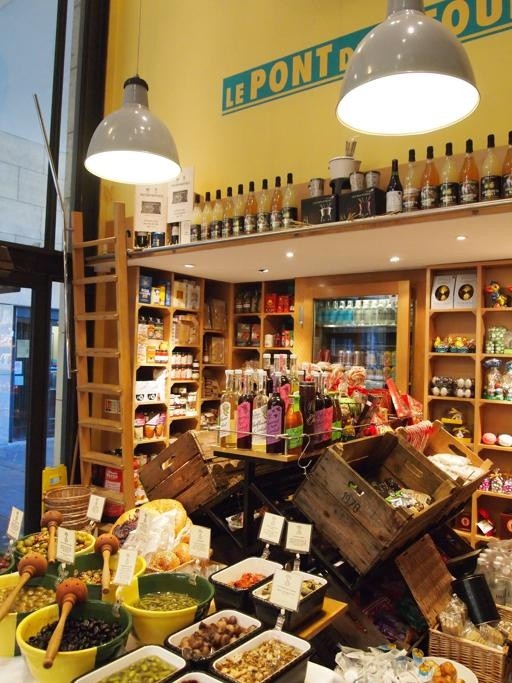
[103,448,125,518]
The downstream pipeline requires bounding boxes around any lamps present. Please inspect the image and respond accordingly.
[82,1,181,187]
[334,1,481,138]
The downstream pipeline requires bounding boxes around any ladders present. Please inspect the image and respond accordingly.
[71,202,135,536]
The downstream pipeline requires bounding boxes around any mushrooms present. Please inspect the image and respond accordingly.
[179,616,258,657]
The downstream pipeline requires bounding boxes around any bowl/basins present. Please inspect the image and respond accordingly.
[42,498,93,532]
[74,644,188,683]
[209,556,285,616]
[251,571,327,625]
[172,670,225,683]
[163,608,262,670]
[212,629,312,683]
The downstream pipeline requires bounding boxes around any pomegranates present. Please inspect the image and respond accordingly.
[173,543,193,564]
[151,550,181,571]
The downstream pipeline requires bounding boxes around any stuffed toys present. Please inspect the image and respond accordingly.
[484,281,508,308]
[442,407,472,444]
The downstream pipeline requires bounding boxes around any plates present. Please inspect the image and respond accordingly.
[412,655,481,683]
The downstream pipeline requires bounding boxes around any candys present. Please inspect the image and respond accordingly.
[299,360,365,399]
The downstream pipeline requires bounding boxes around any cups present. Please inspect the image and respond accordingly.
[349,170,380,193]
[328,157,361,180]
[306,176,325,198]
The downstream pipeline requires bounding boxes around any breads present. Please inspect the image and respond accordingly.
[429,659,465,683]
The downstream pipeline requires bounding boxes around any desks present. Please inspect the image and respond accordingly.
[0,560,349,683]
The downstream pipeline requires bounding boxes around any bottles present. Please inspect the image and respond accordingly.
[318,299,397,324]
[170,222,179,245]
[451,545,512,627]
[191,172,298,241]
[384,133,512,210]
[136,231,165,247]
[217,352,341,454]
[134,384,197,442]
[172,349,200,381]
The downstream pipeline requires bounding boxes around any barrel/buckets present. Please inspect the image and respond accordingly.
[58,551,147,602]
[15,600,134,682]
[0,572,63,658]
[9,529,98,575]
[115,572,216,646]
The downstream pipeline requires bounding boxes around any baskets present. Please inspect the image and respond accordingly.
[43,483,92,529]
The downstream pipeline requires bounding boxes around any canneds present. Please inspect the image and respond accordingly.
[170,387,187,417]
[171,352,199,380]
[146,345,156,363]
[154,349,168,364]
[338,350,396,388]
